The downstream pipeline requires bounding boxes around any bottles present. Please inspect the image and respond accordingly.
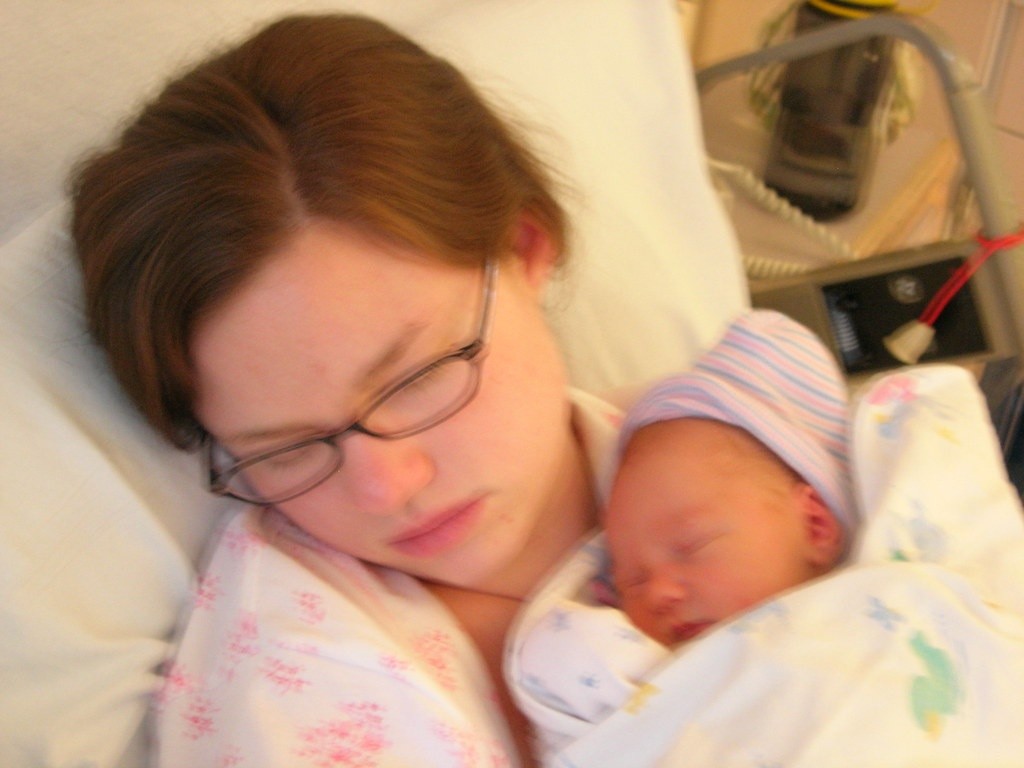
[763,0,938,221]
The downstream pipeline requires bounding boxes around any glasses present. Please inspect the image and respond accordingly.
[199,243,495,505]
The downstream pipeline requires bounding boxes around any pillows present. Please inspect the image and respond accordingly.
[0,0,754,768]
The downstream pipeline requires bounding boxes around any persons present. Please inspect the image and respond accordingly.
[502,310,1024,768]
[69,10,629,768]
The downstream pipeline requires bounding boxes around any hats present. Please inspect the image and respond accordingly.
[617,309,861,542]
